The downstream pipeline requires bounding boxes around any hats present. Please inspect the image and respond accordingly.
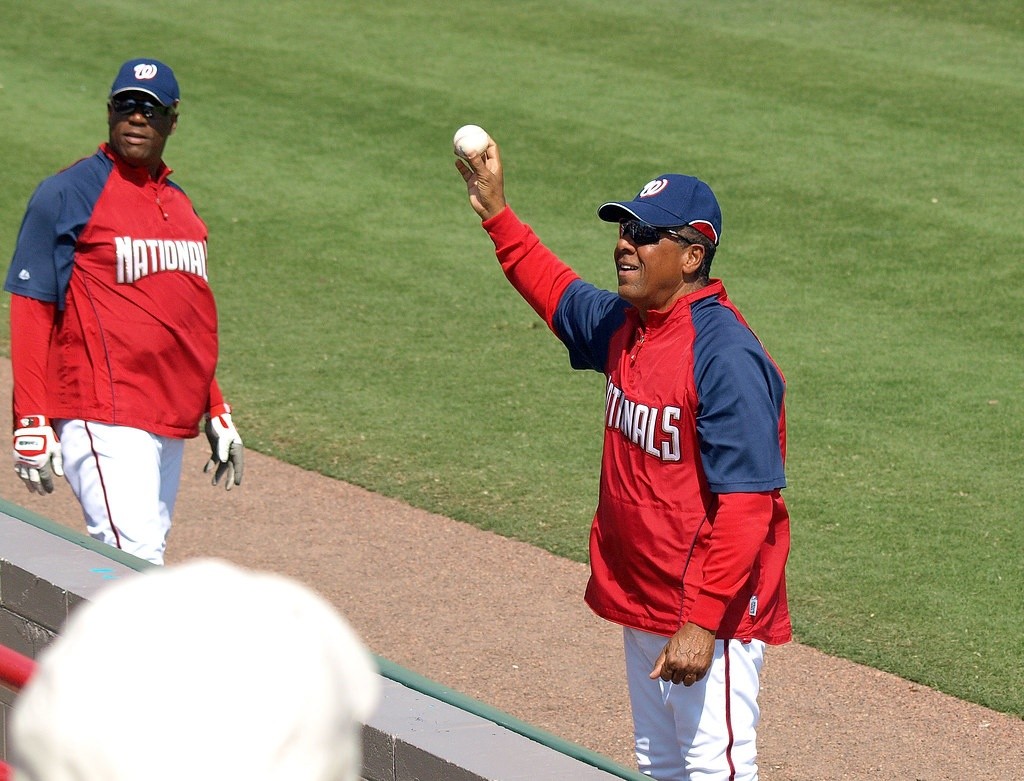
[109,58,180,108]
[597,174,721,247]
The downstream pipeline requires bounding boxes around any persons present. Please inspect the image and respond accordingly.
[453,133,793,781]
[3,59,242,563]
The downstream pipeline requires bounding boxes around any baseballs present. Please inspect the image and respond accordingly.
[450,124,491,161]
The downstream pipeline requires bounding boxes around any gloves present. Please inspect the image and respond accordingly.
[203,403,245,491]
[12,415,64,496]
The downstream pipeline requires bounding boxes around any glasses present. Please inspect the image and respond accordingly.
[112,98,172,120]
[619,218,693,245]
